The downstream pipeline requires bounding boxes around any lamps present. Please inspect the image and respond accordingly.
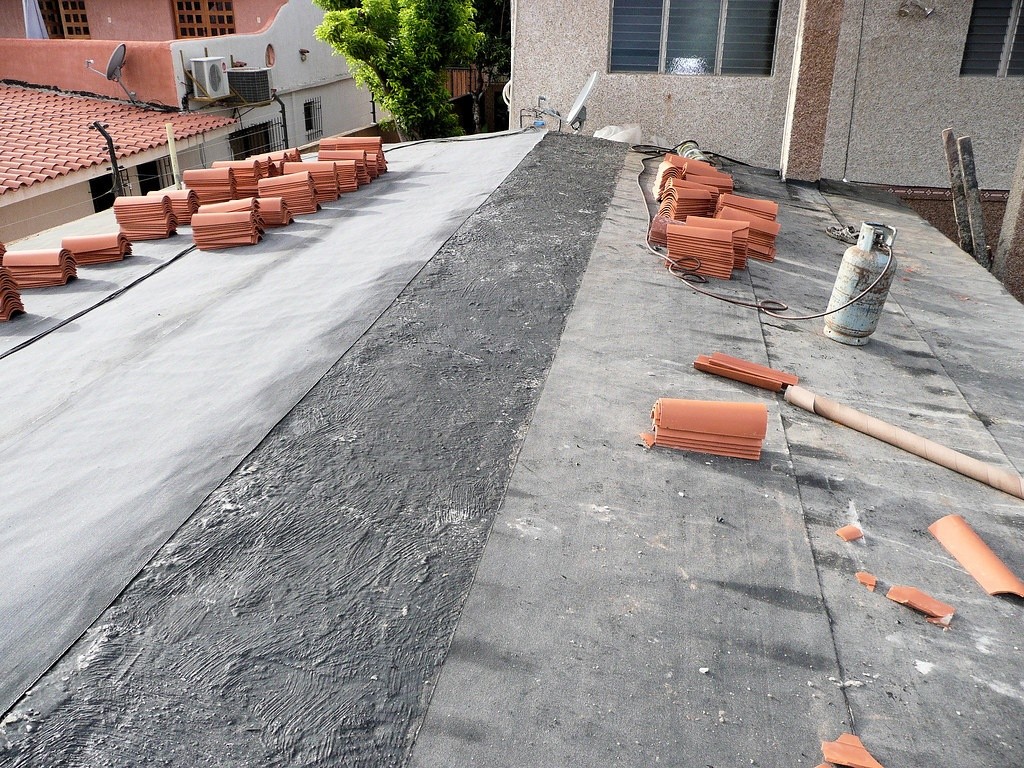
[899,0,937,20]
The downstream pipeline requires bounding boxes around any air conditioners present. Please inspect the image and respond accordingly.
[189,56,230,99]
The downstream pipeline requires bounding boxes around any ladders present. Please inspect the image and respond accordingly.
[941,128,989,268]
